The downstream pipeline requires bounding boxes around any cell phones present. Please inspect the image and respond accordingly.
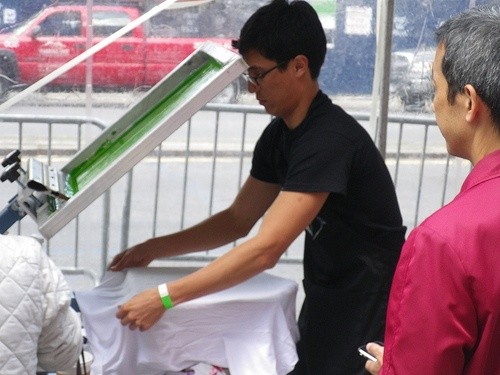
[357,340,384,362]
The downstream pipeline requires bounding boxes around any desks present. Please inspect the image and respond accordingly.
[74,267,302,375]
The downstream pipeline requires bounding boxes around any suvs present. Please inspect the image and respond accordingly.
[391,43,442,111]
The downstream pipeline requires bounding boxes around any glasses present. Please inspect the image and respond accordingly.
[242,58,291,88]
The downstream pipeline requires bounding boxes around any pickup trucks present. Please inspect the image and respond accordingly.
[0,5,254,105]
